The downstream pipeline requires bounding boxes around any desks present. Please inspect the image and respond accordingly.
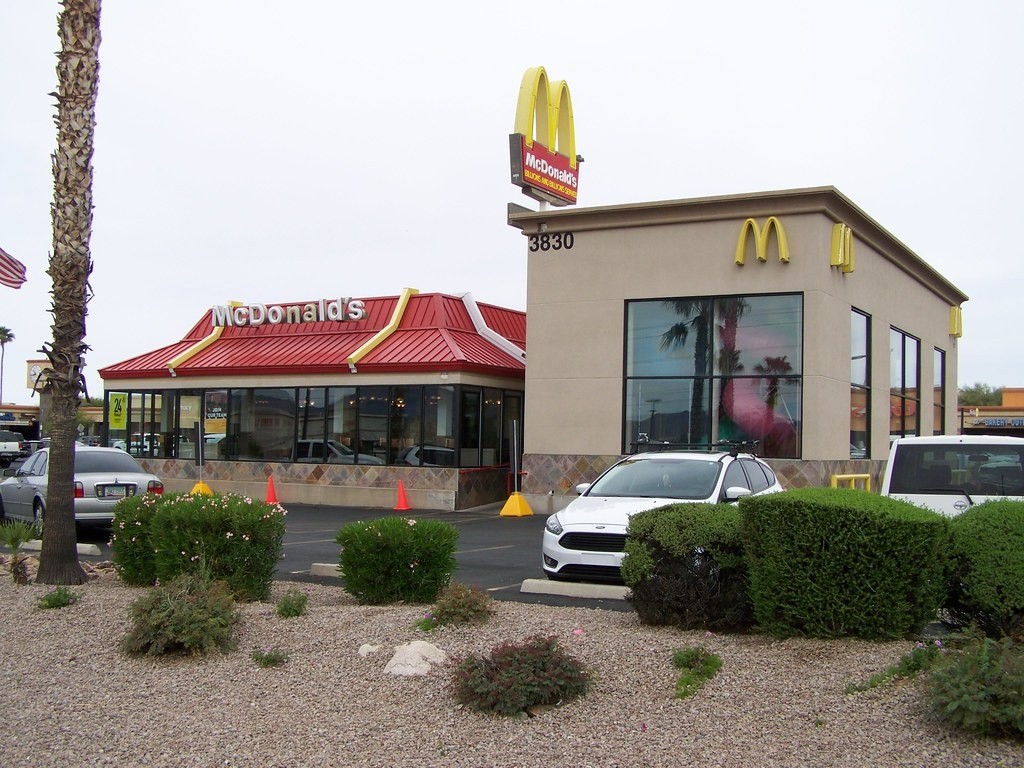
[373,451,386,458]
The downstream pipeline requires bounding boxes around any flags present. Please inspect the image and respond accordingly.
[0,247,27,289]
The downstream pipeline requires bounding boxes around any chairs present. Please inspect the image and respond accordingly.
[918,465,961,491]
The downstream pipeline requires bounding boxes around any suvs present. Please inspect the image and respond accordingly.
[0,430,28,469]
[290,438,386,465]
[113,432,160,456]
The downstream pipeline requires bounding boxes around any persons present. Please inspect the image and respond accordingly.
[207,395,215,413]
[88,440,93,446]
[93,438,99,446]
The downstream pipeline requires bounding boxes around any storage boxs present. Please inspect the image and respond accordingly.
[342,438,351,446]
[280,448,292,458]
[156,434,168,443]
[460,448,497,467]
[444,438,456,448]
[403,439,417,448]
[379,438,403,448]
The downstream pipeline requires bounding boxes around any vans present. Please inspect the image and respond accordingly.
[880,435,1024,517]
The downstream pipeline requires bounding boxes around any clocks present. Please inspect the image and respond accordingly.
[30,365,42,384]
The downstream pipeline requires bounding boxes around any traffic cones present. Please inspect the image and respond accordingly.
[393,480,413,511]
[263,474,279,505]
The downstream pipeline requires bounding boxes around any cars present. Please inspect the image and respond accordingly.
[396,443,455,468]
[0,446,166,537]
[542,438,786,584]
[36,437,89,453]
